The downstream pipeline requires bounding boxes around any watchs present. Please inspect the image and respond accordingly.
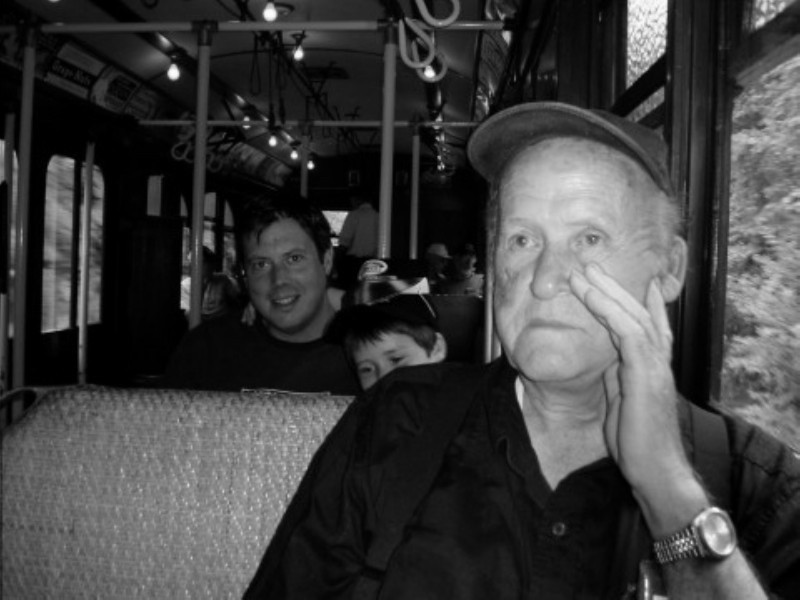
[652,507,738,565]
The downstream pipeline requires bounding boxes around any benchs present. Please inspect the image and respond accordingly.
[0,386,351,600]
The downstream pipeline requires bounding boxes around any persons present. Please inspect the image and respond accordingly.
[163,101,800,600]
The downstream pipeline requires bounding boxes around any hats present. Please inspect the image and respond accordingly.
[466,100,675,202]
